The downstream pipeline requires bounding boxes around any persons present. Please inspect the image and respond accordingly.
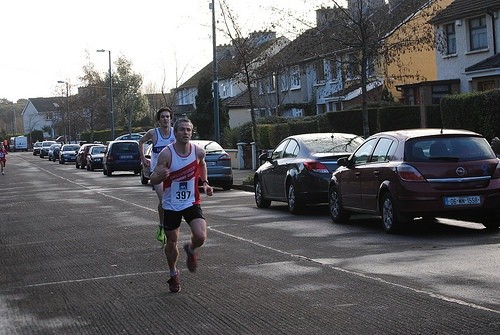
[139,107,177,244]
[0,139,8,176]
[150,117,213,293]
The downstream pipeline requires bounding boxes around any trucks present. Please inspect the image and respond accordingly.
[8,136,28,152]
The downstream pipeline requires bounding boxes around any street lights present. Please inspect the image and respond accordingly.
[97,49,114,141]
[12,127,14,136]
[57,81,71,144]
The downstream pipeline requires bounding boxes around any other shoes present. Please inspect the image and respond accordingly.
[166,268,181,292]
[183,242,198,272]
[156,224,167,244]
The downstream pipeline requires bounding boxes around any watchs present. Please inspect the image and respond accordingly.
[203,180,209,184]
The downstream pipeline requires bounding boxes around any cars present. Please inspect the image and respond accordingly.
[47,144,61,161]
[86,146,105,171]
[58,144,82,164]
[40,140,57,158]
[74,144,102,169]
[189,140,233,191]
[326,128,500,233]
[116,133,143,142]
[100,140,142,176]
[140,144,153,184]
[253,133,365,214]
[32,142,42,155]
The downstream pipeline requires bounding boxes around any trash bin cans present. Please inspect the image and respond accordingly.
[243,146,252,169]
